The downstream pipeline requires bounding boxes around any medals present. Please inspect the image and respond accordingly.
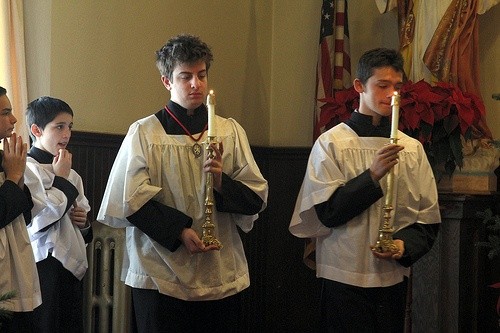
[192,142,203,158]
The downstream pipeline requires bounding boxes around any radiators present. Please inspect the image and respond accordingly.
[83,222,132,333]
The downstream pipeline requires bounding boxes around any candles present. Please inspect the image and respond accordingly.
[390,91,400,139]
[207,90,216,136]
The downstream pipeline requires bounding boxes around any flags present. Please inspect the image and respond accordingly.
[313,0,353,142]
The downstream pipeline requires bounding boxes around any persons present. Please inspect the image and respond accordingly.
[288,47,443,332]
[18,97,92,333]
[97,35,269,333]
[0,85,42,333]
